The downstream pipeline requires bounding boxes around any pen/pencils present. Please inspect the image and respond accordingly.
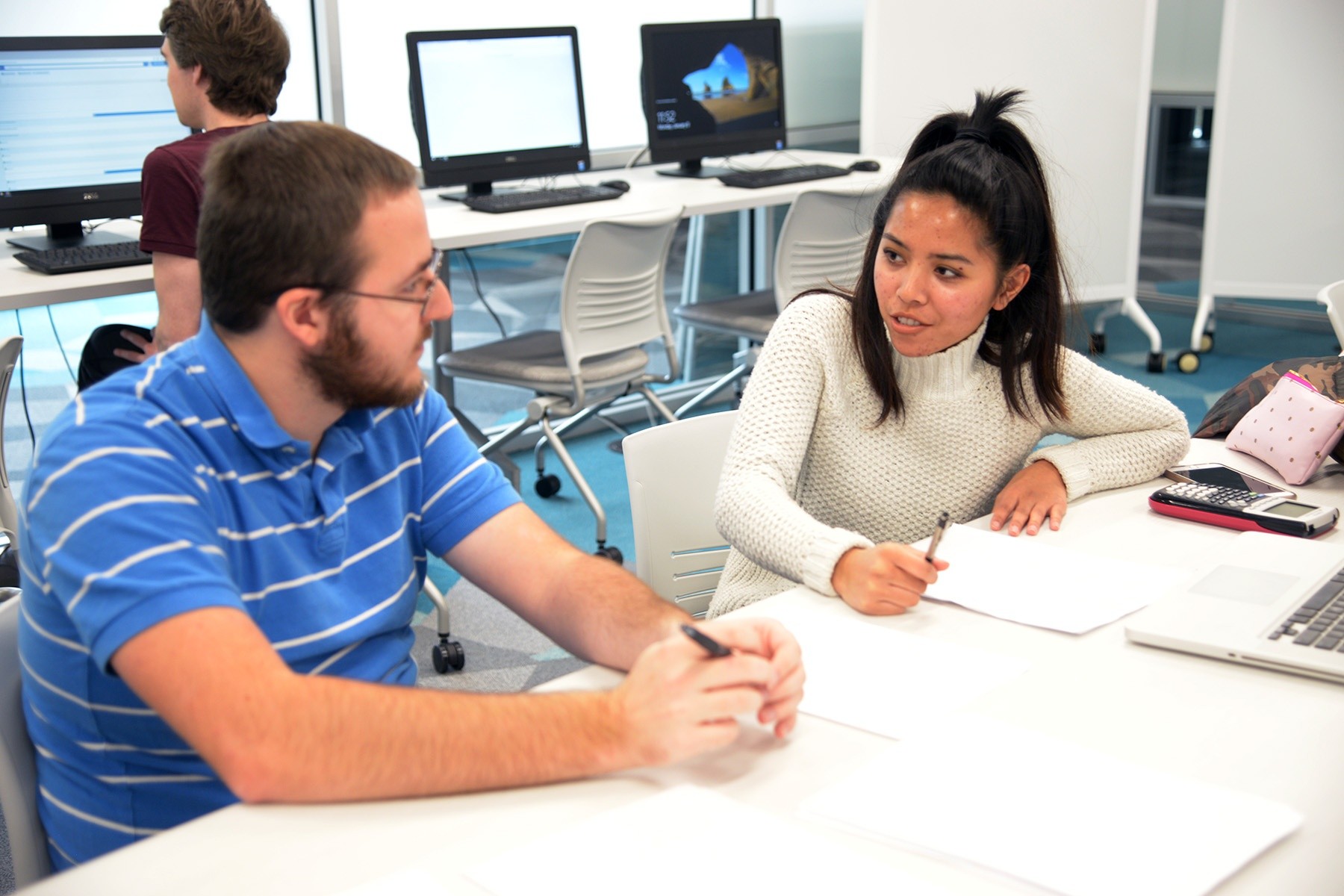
[682,628,731,656]
[925,512,950,564]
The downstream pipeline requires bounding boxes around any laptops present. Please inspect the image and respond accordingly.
[1124,531,1344,684]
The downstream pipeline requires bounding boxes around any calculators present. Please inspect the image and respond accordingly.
[1148,482,1340,538]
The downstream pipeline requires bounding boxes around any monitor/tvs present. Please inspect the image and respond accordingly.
[405,26,591,201]
[0,36,205,250]
[640,18,786,179]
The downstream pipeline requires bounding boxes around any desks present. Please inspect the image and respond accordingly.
[0,436,1344,895]
[1,149,902,497]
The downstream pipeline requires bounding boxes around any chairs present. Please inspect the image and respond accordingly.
[1317,279,1344,350]
[621,411,738,621]
[0,593,54,891]
[438,205,686,567]
[674,172,893,418]
[0,335,25,587]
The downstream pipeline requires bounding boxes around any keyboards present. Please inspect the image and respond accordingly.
[14,240,153,275]
[718,164,850,188]
[463,184,624,214]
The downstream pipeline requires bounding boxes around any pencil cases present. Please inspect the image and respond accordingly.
[1225,369,1344,485]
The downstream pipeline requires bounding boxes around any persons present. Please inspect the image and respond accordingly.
[705,88,1190,622]
[20,122,804,876]
[78,0,291,394]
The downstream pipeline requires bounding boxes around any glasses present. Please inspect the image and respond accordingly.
[271,247,445,318]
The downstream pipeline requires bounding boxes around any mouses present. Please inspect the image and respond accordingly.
[849,161,881,171]
[599,180,630,192]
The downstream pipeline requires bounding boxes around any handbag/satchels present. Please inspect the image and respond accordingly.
[1224,369,1344,485]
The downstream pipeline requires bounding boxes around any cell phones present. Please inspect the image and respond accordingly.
[1163,462,1297,501]
